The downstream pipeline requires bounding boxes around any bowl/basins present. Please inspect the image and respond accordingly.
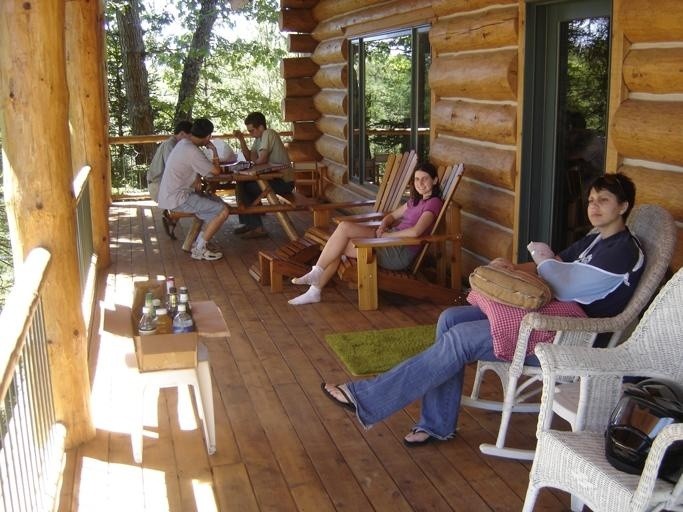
[220,165,235,173]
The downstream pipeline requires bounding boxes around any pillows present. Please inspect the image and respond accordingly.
[469,263,551,310]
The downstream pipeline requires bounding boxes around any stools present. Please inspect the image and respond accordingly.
[125,343,217,463]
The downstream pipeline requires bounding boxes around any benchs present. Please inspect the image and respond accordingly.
[170,193,317,252]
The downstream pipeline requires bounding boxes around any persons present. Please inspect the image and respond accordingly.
[158,118,230,261]
[286,162,443,306]
[233,112,294,240]
[322,172,647,447]
[147,122,193,240]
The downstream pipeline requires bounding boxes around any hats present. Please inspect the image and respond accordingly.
[190,119,213,136]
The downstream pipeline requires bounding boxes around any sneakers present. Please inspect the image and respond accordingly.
[233,224,268,239]
[190,241,223,261]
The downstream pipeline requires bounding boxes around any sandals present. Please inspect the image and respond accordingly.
[162,209,176,240]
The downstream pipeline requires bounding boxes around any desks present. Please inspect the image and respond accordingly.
[181,168,300,252]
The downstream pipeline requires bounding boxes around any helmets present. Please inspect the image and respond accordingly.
[604,379,682,476]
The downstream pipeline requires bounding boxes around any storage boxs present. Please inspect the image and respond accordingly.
[130,279,230,372]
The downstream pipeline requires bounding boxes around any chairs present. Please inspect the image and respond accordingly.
[344,162,465,310]
[248,150,419,294]
[522,266,682,511]
[460,204,676,460]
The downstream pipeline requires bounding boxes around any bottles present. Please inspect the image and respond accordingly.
[139,276,193,334]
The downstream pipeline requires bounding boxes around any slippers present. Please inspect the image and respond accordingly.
[320,383,356,413]
[404,429,434,447]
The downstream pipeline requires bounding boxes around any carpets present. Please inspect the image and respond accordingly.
[325,325,435,376]
